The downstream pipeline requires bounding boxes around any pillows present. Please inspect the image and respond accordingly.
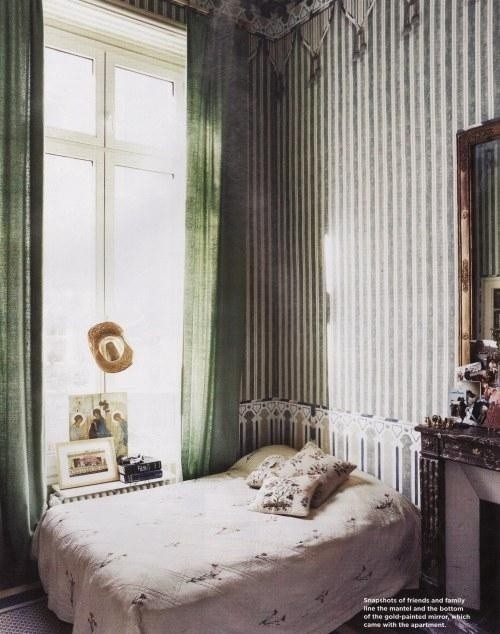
[245,442,357,521]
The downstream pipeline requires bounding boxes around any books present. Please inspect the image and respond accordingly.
[117,455,162,475]
[119,469,163,483]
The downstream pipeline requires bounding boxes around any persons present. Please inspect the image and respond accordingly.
[70,414,86,440]
[114,412,128,447]
[88,408,112,438]
[458,358,500,411]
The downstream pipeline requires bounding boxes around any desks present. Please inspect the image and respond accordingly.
[49,472,170,506]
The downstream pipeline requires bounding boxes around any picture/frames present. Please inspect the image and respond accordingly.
[477,274,499,338]
[57,435,120,490]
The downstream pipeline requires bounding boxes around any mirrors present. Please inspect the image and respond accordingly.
[457,116,499,383]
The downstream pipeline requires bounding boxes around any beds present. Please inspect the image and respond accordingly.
[28,443,417,634]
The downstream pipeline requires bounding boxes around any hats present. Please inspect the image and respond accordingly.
[89,322,132,374]
